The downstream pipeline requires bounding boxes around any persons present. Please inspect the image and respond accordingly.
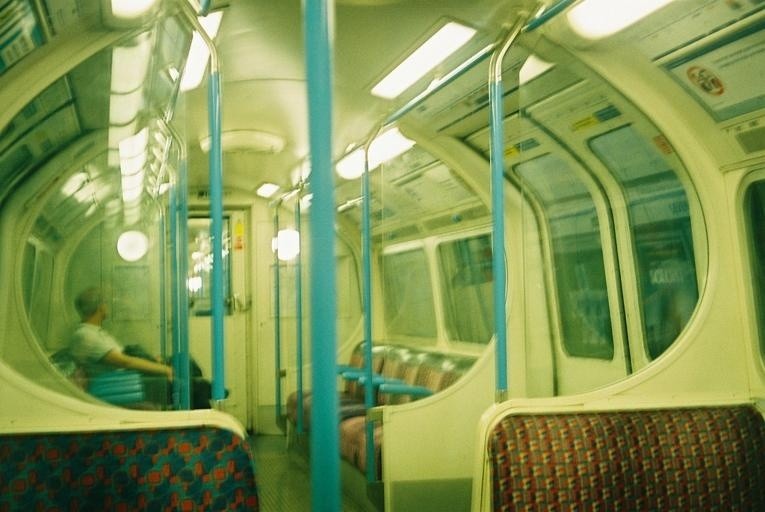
[70,287,231,410]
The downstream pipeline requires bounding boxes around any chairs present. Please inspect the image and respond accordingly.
[49,347,174,411]
[287,341,479,480]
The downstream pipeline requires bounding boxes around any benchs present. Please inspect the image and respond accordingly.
[0,420,258,512]
[477,401,765,512]
